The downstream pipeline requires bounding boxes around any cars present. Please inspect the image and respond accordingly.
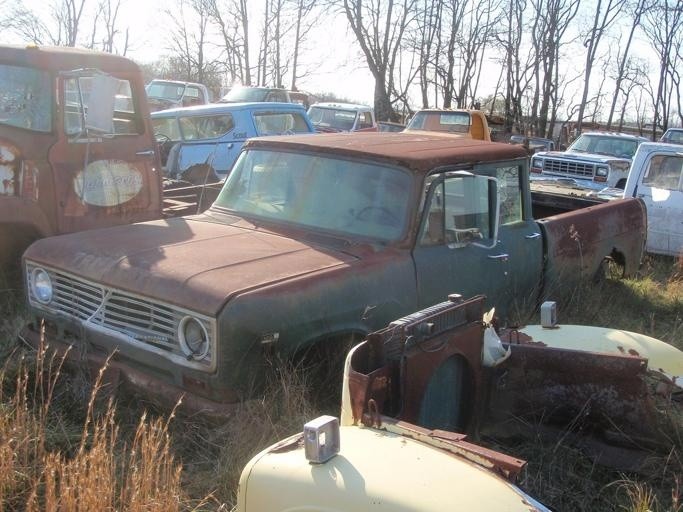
[628,142,683,258]
[149,103,320,188]
[1,44,682,512]
[235,288,683,512]
[526,131,654,193]
[402,107,543,157]
[21,131,647,420]
[306,102,381,134]
[0,44,163,257]
[659,128,683,146]
[135,77,210,114]
[510,135,554,155]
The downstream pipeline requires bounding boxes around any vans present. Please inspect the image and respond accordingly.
[211,86,308,131]
